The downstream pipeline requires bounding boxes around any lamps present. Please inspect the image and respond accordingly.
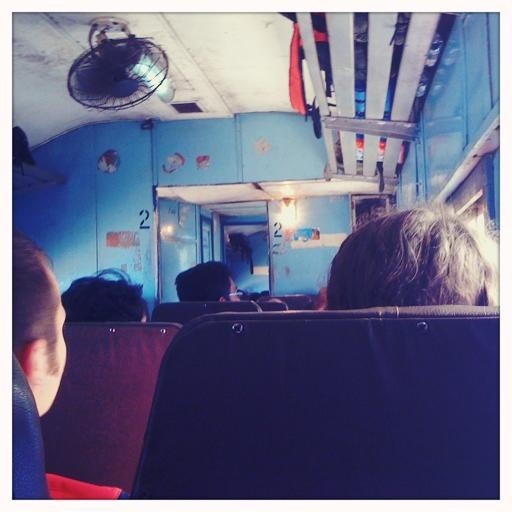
[102,27,177,104]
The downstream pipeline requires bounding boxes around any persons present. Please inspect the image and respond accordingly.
[175,261,242,302]
[12,233,130,500]
[61,276,149,322]
[326,203,500,310]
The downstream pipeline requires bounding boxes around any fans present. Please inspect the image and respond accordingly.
[68,17,170,112]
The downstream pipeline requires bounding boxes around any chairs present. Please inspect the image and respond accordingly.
[13,294,499,499]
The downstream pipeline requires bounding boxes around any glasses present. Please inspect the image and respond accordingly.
[228,290,245,297]
